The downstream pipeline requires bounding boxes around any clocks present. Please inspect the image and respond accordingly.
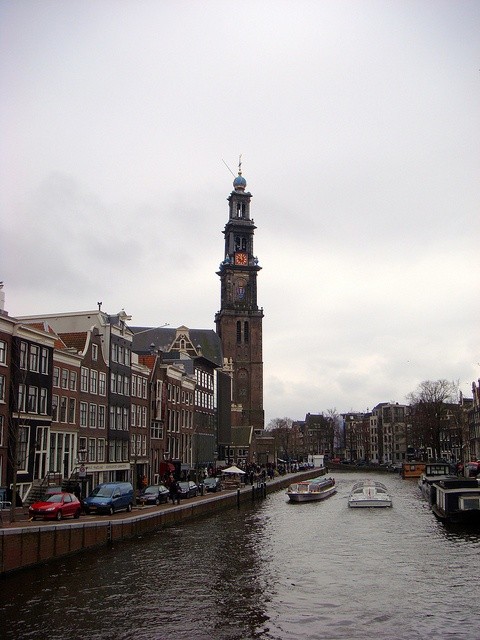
[235,251,249,264]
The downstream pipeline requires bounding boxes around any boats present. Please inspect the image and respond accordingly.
[418,463,458,502]
[432,476,480,523]
[348,479,393,507]
[284,477,338,501]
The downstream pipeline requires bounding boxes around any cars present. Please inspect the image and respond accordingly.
[136,485,170,505]
[29,492,81,520]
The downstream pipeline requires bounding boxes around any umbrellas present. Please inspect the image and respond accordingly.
[221,465,247,474]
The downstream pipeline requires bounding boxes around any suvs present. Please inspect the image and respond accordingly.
[203,478,217,493]
[176,480,199,498]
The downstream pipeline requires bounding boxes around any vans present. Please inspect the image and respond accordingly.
[83,481,134,515]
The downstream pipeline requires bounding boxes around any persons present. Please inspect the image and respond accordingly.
[74,483,81,501]
[180,466,220,484]
[140,474,148,488]
[169,473,178,504]
